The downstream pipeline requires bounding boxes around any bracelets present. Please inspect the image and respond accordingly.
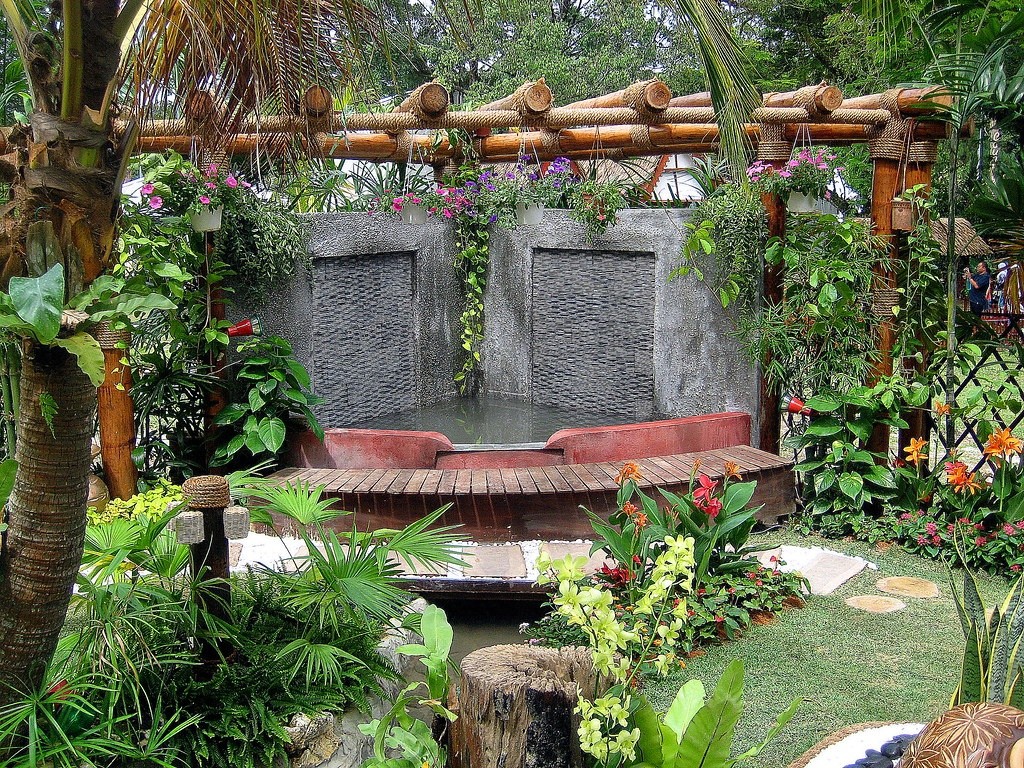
[968,277,972,280]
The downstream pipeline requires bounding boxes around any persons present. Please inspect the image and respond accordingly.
[966,261,1007,319]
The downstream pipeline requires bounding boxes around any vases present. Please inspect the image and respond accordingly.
[187,204,224,233]
[787,185,820,212]
[401,203,427,224]
[583,191,605,213]
[516,201,544,226]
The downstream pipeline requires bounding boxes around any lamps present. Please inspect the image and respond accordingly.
[778,390,811,415]
[227,314,263,338]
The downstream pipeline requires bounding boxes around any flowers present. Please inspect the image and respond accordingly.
[458,154,580,235]
[140,162,251,224]
[746,144,846,202]
[569,176,630,244]
[367,184,470,223]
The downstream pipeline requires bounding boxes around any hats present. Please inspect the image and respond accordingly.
[998,263,1006,269]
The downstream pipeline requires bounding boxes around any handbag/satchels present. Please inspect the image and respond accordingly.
[983,298,988,310]
